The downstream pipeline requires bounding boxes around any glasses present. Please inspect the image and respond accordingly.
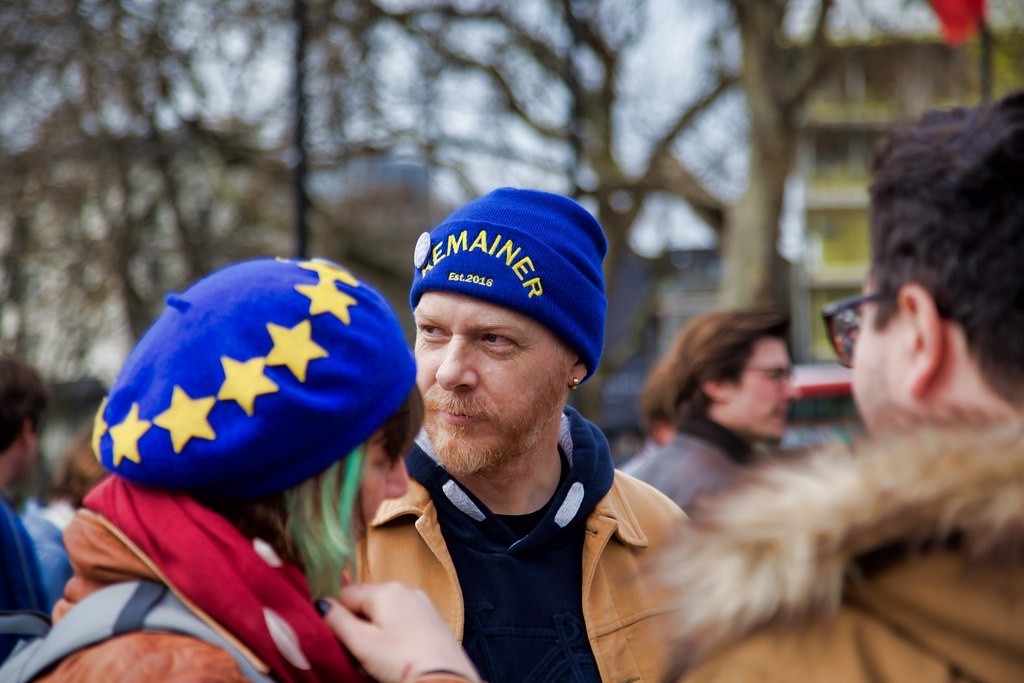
[744,365,794,382]
[822,272,966,367]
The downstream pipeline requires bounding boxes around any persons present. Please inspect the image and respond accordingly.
[361,186,690,683]
[1,256,483,682]
[0,304,795,682]
[657,88,1023,683]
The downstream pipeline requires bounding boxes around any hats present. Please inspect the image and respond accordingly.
[409,187,608,383]
[91,256,417,496]
